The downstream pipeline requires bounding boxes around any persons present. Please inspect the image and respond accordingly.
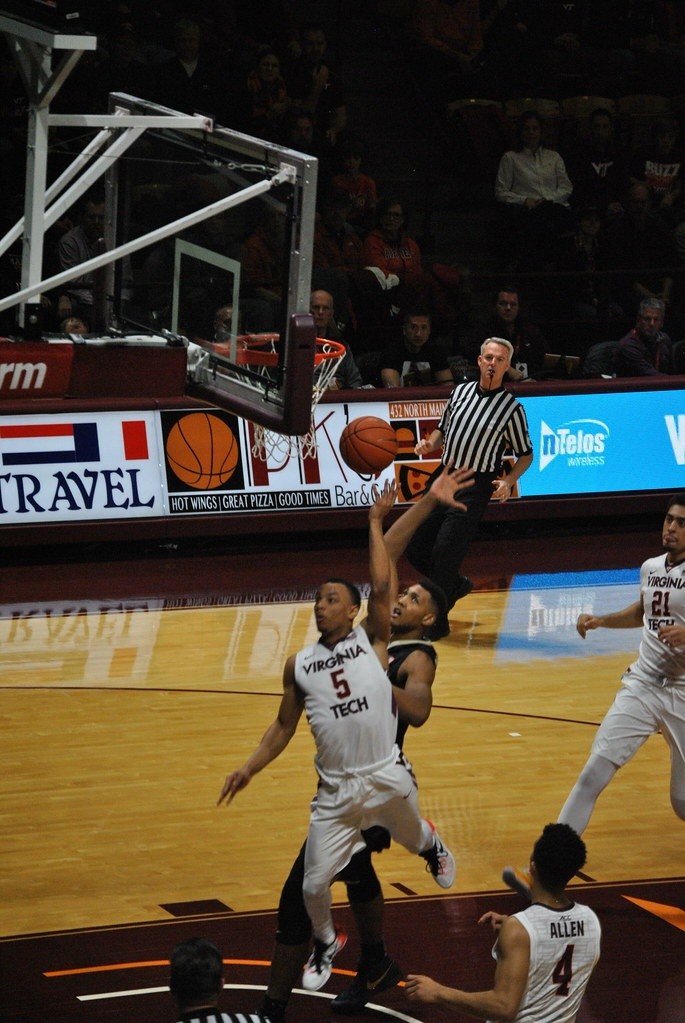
[405,337,533,642]
[502,493,685,900]
[216,479,456,991]
[406,822,602,1023]
[170,935,274,1023]
[258,458,475,1023]
[0,0,685,391]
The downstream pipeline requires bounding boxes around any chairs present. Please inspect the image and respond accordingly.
[379,0,685,222]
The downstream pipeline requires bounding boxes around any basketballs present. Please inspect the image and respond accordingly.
[339,416,398,475]
[166,412,239,489]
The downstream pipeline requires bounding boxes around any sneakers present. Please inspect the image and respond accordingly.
[248,995,293,1023]
[501,862,539,903]
[326,952,403,1017]
[301,923,350,991]
[421,818,455,890]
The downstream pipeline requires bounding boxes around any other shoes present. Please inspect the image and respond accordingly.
[446,576,473,615]
[428,622,450,643]
